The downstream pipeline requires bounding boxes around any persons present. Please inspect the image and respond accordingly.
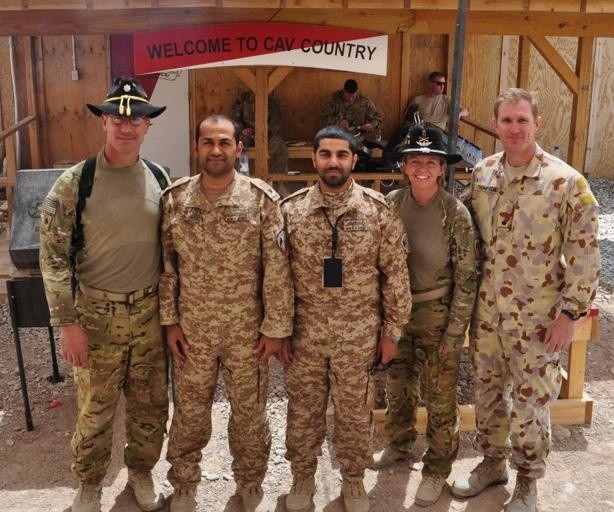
[405,71,469,133]
[273,126,412,512]
[232,90,288,174]
[368,122,479,507]
[39,78,173,512]
[156,116,293,512]
[452,88,601,511]
[319,79,382,171]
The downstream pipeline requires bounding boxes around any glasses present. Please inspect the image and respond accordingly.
[103,112,148,126]
[431,80,446,86]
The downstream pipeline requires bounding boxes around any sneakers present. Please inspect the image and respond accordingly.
[415,474,446,507]
[286,477,315,512]
[236,483,275,512]
[365,446,417,468]
[128,468,165,511]
[72,481,102,512]
[505,473,537,512]
[341,478,370,512]
[451,456,508,499]
[170,483,197,512]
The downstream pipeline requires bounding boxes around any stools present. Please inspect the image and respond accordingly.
[370,307,600,436]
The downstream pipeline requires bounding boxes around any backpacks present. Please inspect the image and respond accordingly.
[70,156,172,302]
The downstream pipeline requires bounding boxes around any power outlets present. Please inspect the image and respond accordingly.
[71,70,78,81]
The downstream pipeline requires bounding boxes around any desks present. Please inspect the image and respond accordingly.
[7,267,63,431]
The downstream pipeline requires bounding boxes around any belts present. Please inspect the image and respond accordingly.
[78,281,157,304]
[411,286,450,303]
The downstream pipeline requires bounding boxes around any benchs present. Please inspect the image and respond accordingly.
[245,139,382,159]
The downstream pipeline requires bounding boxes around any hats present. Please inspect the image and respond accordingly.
[86,76,167,118]
[385,127,463,165]
